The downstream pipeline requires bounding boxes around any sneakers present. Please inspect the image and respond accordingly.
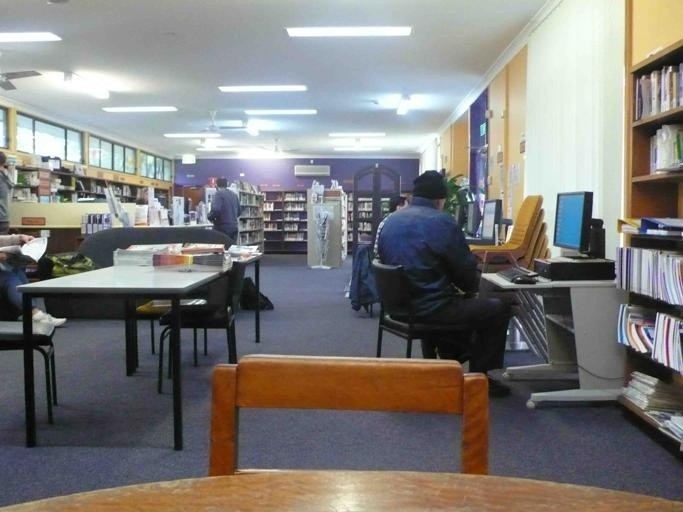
[489,379,511,397]
[18,310,67,328]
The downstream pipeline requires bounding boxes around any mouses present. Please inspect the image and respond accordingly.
[512,275,537,284]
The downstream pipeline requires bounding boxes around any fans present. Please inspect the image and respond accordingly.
[0,70,43,90]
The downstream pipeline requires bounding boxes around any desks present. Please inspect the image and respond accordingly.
[481,266,632,410]
[0,468,682,512]
[16,250,264,451]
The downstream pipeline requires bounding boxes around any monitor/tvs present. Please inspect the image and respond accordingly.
[553,191,605,259]
[454,205,465,227]
[466,202,481,235]
[481,199,513,241]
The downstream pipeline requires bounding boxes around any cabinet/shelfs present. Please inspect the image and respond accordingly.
[379,195,392,218]
[1,163,78,203]
[355,195,373,242]
[204,187,265,255]
[617,1,683,454]
[347,192,355,253]
[283,191,308,254]
[260,191,283,254]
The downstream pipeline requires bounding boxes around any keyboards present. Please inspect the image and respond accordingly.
[496,266,531,282]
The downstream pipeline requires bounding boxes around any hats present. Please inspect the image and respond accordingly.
[413,170,447,198]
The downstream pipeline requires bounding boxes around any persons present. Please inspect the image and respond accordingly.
[208,178,240,243]
[0,233,67,328]
[0,151,15,233]
[372,196,410,322]
[379,170,509,397]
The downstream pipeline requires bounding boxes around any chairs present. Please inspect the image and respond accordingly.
[0,321,57,422]
[137,246,224,367]
[370,257,467,358]
[158,262,247,393]
[353,243,381,317]
[467,194,547,362]
[209,354,489,475]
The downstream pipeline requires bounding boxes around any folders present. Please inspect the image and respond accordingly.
[641,218,683,236]
[617,218,640,234]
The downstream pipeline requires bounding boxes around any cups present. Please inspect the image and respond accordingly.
[188,211,198,225]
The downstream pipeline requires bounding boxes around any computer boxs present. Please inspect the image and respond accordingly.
[534,257,616,281]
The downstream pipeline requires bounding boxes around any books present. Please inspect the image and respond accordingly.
[616,63,683,449]
[113,242,264,273]
[237,193,307,253]
[0,237,48,263]
[347,196,391,252]
